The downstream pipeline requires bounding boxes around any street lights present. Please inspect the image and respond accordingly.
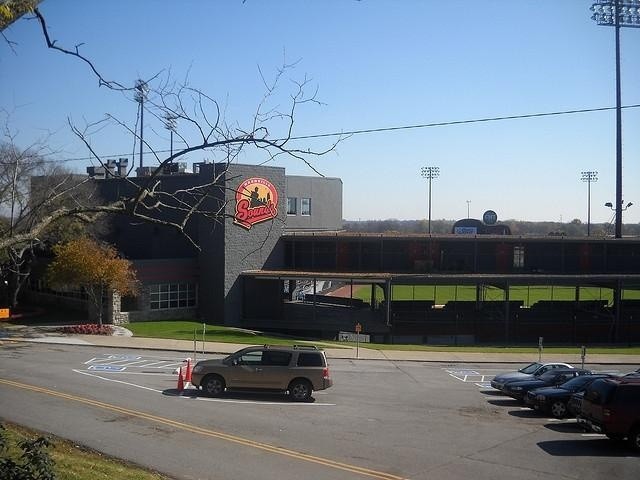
[581,172,598,236]
[590,0,640,238]
[421,166,439,233]
[135,78,148,168]
[165,115,177,164]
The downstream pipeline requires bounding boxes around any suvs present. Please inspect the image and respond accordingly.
[191,343,332,401]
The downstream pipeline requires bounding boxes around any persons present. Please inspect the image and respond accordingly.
[250,187,260,207]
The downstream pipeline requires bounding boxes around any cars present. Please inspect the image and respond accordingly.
[491,361,640,442]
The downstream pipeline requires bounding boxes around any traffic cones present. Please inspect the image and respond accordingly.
[178,368,183,392]
[184,359,192,381]
